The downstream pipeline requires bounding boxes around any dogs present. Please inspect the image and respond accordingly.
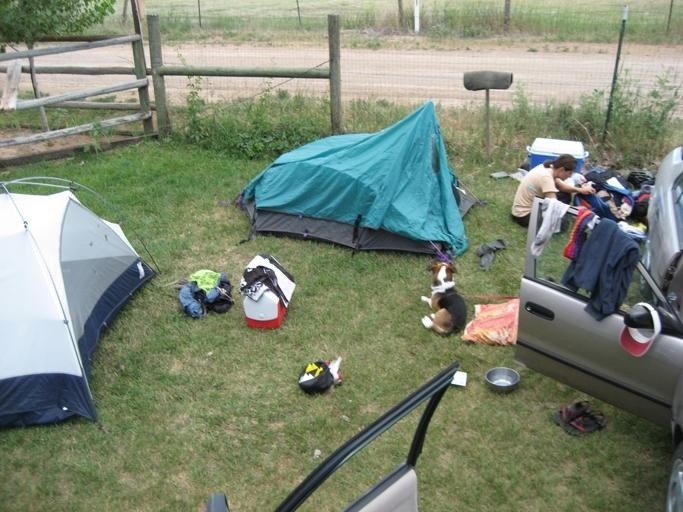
[421,260,467,338]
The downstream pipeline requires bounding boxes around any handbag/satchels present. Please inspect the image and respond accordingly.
[573,171,634,223]
[618,302,660,358]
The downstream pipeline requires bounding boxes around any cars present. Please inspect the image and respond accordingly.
[517,146,682,509]
[198,362,465,512]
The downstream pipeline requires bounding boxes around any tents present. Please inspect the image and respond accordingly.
[237,99,488,266]
[0,175,162,440]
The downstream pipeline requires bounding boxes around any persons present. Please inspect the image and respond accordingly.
[511,153,598,228]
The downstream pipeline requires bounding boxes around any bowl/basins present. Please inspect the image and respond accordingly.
[484,367,520,391]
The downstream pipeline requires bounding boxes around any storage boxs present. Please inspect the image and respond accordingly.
[527,137,588,173]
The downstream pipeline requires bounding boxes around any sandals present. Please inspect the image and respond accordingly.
[564,410,608,435]
[552,401,590,425]
